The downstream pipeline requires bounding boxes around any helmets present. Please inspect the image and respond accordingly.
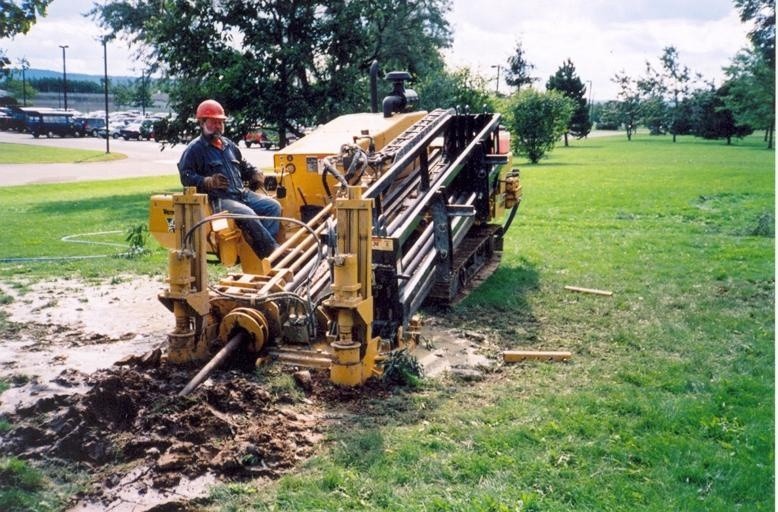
[196,99,227,119]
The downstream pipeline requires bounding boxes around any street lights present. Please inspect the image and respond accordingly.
[59,44,68,124]
[95,32,118,153]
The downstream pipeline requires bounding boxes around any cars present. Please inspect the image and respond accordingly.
[0,101,272,148]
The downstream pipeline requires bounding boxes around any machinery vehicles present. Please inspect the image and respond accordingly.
[145,67,522,399]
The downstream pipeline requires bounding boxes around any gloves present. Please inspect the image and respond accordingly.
[207,172,231,191]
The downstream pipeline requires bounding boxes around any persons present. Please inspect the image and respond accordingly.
[176,99,283,261]
